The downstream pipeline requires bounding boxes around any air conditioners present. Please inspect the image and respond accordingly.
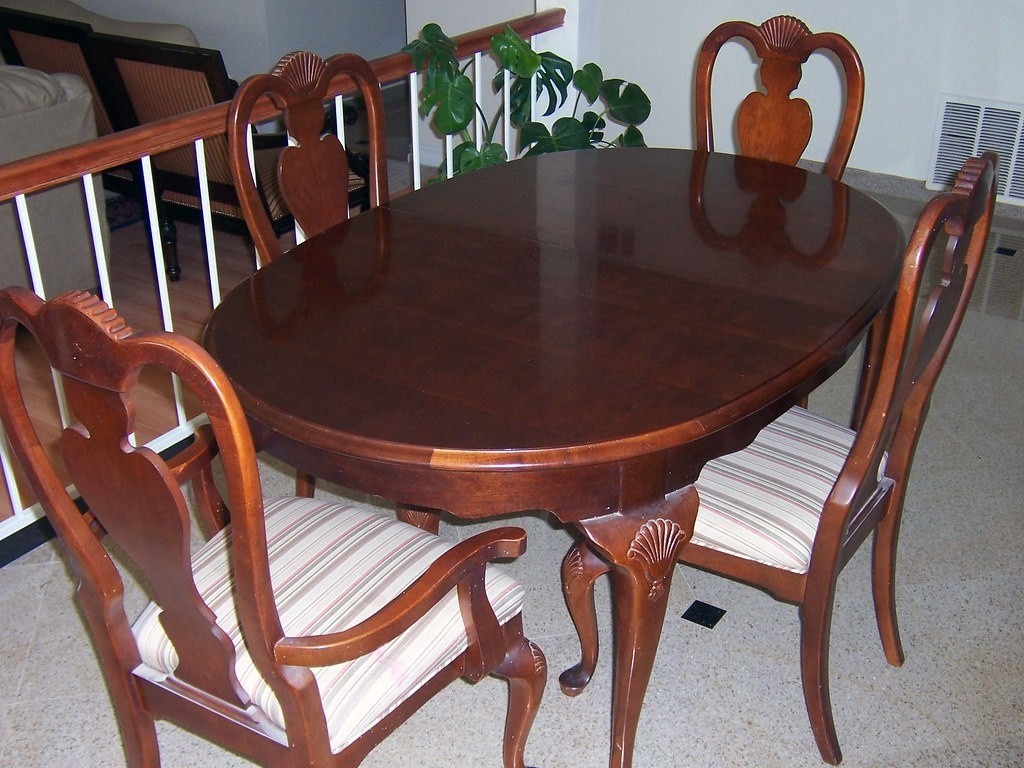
[922,93,1024,206]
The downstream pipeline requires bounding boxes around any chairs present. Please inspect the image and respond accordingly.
[227,51,389,266]
[694,12,865,182]
[0,5,181,276]
[0,285,544,768]
[87,32,373,284]
[560,151,1000,768]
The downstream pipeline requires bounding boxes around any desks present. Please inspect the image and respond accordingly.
[198,148,905,768]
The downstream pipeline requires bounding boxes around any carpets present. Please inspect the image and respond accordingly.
[864,191,1024,320]
